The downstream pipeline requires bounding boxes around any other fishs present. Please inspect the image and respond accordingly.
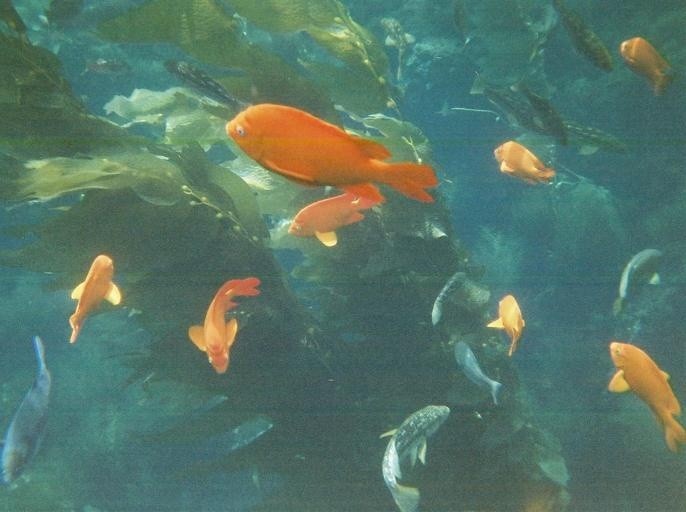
[452,0,670,188]
